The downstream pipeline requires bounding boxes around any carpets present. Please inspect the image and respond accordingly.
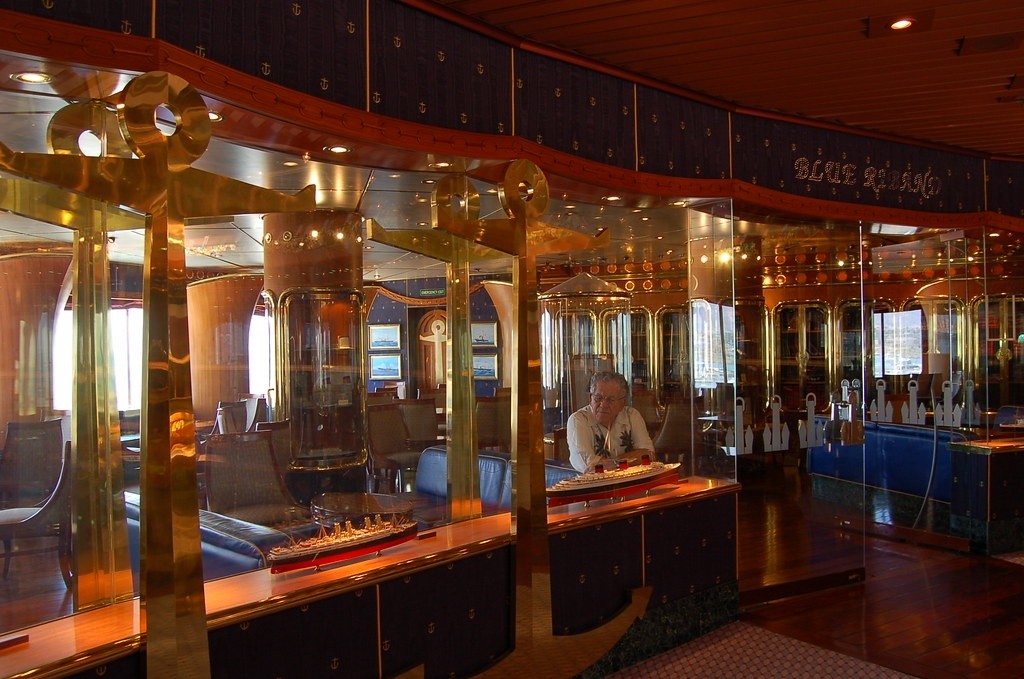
[599,622,923,679]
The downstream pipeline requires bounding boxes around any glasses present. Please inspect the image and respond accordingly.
[592,393,626,405]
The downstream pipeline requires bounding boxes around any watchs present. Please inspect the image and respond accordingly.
[613,460,620,468]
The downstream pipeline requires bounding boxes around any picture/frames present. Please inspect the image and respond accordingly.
[368,324,401,351]
[368,353,402,381]
[473,353,499,380]
[471,321,498,349]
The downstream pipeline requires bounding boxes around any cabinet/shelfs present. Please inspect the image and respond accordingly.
[553,293,1023,396]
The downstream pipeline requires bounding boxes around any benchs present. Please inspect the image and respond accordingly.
[122,492,299,583]
[393,443,588,529]
[804,412,982,551]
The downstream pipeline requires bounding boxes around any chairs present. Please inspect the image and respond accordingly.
[553,426,573,464]
[193,391,310,528]
[629,381,787,470]
[872,394,917,424]
[0,417,74,592]
[362,384,518,494]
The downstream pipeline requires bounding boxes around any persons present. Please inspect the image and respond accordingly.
[567,370,656,473]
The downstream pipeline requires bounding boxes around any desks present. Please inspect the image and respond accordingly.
[308,490,414,530]
[0,475,740,679]
[946,437,1024,557]
